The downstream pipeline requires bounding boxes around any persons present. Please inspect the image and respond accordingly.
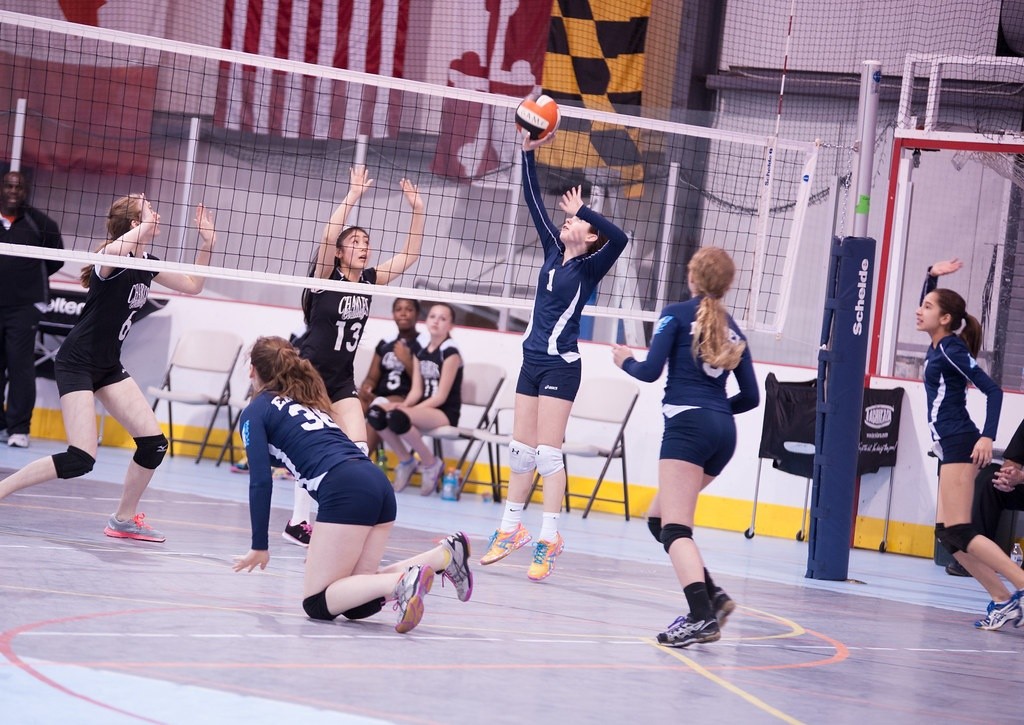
[0,171,64,448]
[916,258,1024,629]
[0,192,217,543]
[480,126,629,581]
[231,336,474,634]
[282,164,423,548]
[367,303,463,496]
[608,244,759,648]
[232,456,249,474]
[946,419,1024,577]
[357,299,421,458]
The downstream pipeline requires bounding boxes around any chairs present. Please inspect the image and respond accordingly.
[146,327,255,469]
[523,377,641,521]
[352,346,518,503]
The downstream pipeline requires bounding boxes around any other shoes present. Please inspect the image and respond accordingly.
[274,469,292,479]
[230,461,249,472]
[946,561,972,576]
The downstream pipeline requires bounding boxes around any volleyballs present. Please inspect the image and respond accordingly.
[514,93,563,140]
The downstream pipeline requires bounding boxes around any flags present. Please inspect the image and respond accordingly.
[0,0,169,176]
[432,0,651,200]
[211,0,410,138]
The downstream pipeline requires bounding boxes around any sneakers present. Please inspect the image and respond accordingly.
[281,520,313,547]
[392,458,417,492]
[0,429,9,442]
[8,433,30,447]
[419,455,445,496]
[381,565,434,633]
[1013,589,1024,628]
[104,512,166,542]
[480,522,532,565]
[436,531,473,602]
[975,594,1021,631]
[527,531,564,582]
[656,613,721,647]
[709,586,734,628]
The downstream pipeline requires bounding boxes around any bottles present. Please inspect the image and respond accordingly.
[376,450,386,477]
[442,465,457,500]
[1010,543,1023,568]
